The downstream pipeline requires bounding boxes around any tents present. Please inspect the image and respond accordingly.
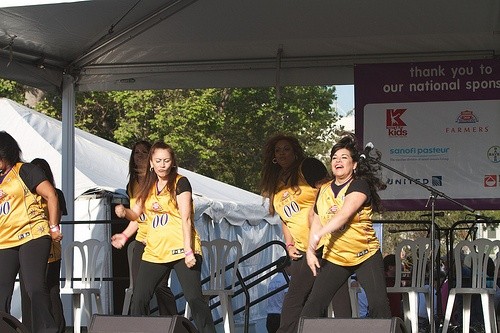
[0,96,384,329]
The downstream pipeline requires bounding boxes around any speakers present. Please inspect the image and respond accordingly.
[0,311,30,333]
[297,316,409,333]
[89,314,197,333]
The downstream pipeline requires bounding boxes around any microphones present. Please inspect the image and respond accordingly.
[388,227,401,233]
[421,212,444,217]
[359,142,374,162]
[470,213,493,221]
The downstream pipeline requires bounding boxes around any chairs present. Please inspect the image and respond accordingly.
[183,239,242,333]
[441,238,500,333]
[387,237,439,333]
[58,239,108,333]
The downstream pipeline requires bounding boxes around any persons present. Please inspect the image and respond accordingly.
[111,141,216,333]
[0,131,68,333]
[266,256,292,333]
[355,249,500,333]
[260,133,391,333]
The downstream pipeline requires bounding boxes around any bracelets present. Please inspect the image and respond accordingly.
[48,224,59,233]
[122,232,128,240]
[285,242,294,249]
[184,249,193,256]
